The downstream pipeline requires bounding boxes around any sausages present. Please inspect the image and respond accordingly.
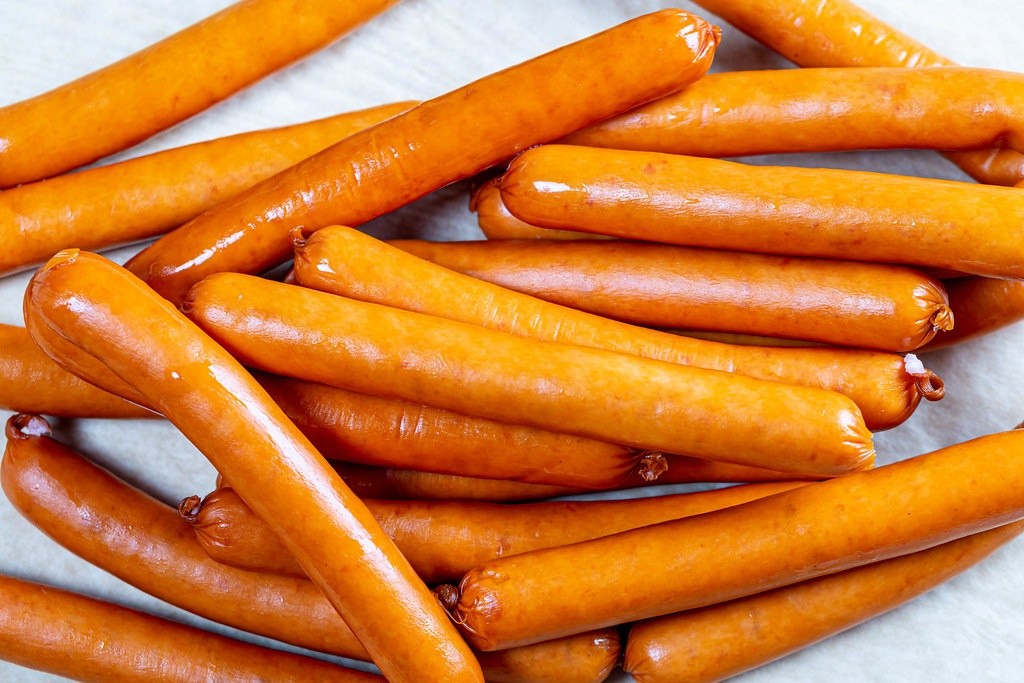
[0,0,1024,683]
